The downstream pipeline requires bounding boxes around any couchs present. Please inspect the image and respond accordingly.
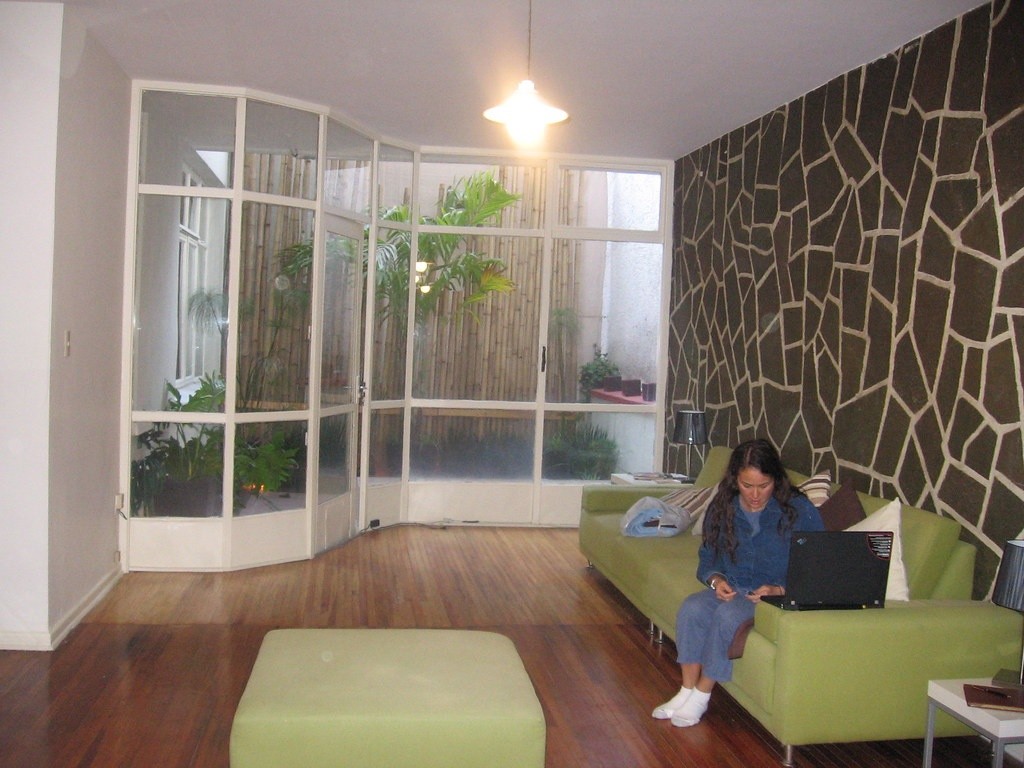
[578,445,1024,768]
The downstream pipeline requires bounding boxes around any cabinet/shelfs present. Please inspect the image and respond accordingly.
[587,387,656,475]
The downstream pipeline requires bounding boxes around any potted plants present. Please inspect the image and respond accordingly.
[130,369,300,518]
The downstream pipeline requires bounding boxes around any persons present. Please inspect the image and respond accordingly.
[650,438,825,729]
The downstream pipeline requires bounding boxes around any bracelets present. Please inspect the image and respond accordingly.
[710,577,720,589]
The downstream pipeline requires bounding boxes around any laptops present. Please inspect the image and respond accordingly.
[760,529,894,610]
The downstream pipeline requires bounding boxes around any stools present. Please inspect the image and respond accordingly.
[229,629,547,768]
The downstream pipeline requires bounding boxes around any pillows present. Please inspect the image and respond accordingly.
[819,476,866,531]
[795,469,832,508]
[842,497,910,602]
[659,487,714,522]
[691,482,719,536]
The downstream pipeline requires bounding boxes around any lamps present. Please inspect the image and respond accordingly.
[482,0,569,125]
[991,538,1024,688]
[672,409,709,484]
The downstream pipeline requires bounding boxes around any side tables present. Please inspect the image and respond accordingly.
[610,473,694,488]
[922,677,1024,768]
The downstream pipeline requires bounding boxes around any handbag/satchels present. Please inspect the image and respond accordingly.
[619,496,692,539]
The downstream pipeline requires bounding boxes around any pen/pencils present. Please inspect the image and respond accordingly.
[971,685,1012,698]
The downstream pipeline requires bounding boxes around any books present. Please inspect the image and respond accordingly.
[963,683,1024,711]
